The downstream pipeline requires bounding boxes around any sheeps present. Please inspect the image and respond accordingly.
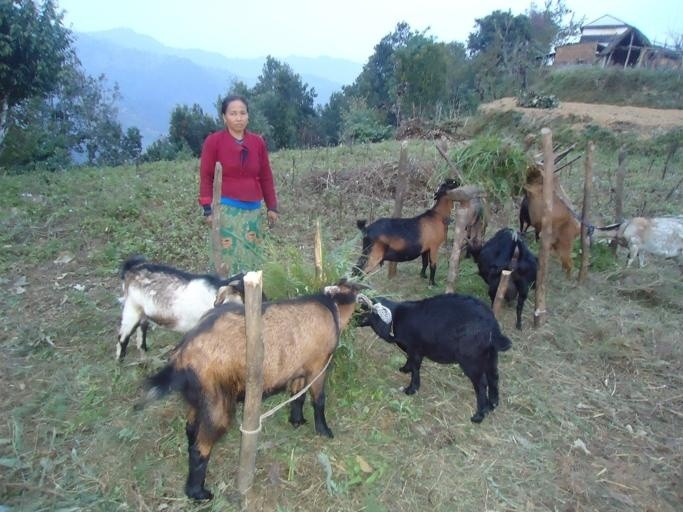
[355,292,513,425]
[143,274,374,502]
[461,228,540,331]
[524,159,580,280]
[350,179,462,291]
[114,253,269,367]
[586,217,683,270]
[520,197,531,232]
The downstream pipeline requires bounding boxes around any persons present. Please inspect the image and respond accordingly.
[197,94,279,276]
